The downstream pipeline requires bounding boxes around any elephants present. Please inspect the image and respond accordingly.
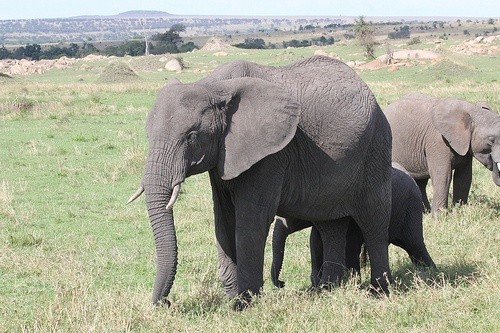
[125,55,392,312]
[385,91,500,217]
[271,162,443,298]
